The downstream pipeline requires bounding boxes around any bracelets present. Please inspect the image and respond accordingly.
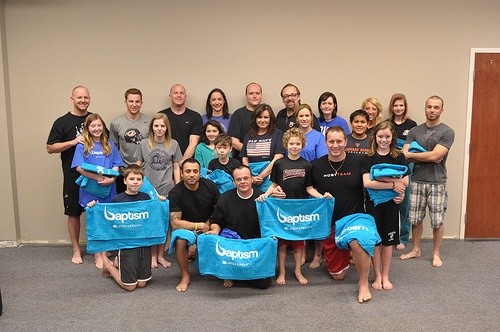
[392,182,395,190]
[195,222,197,231]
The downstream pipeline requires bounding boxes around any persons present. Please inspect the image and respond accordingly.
[168,157,222,292]
[46,83,417,303]
[71,114,124,269]
[401,95,455,267]
[254,128,333,285]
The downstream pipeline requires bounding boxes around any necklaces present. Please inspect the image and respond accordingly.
[328,152,346,174]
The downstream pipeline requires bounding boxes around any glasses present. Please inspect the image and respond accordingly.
[282,93,299,99]
